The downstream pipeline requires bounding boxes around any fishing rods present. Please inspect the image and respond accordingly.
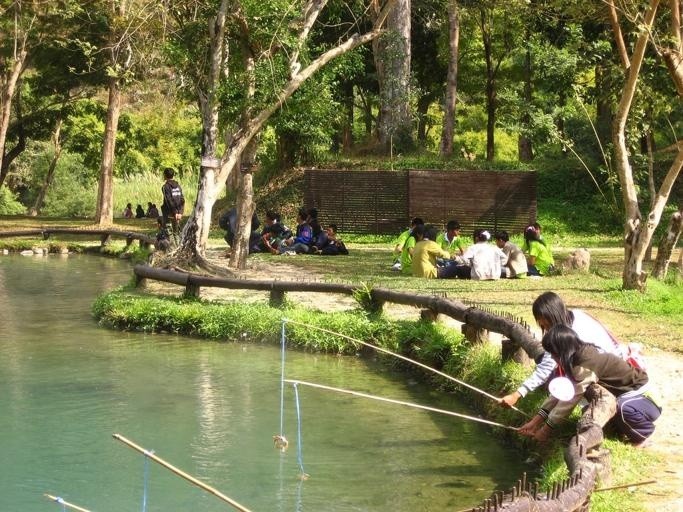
[282,378,538,437]
[46,493,92,512]
[282,317,531,419]
[114,433,251,512]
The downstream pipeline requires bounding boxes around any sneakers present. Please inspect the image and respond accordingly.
[312,245,317,251]
[390,262,402,271]
[314,250,322,255]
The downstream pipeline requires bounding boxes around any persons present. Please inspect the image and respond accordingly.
[214,203,348,256]
[158,167,184,236]
[512,323,662,450]
[123,200,159,220]
[491,291,627,407]
[388,215,556,280]
